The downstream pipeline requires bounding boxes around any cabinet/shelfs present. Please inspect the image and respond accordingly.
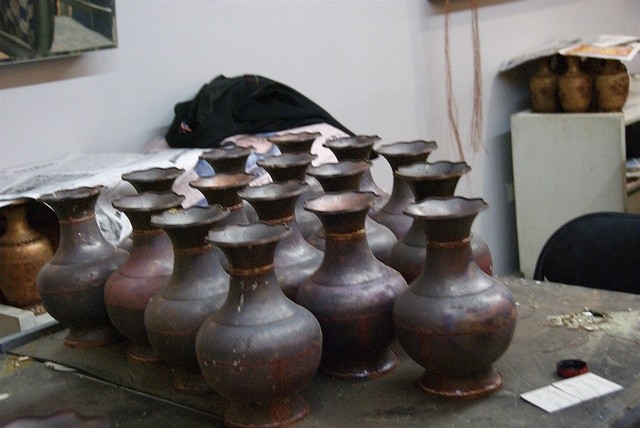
[510,82,640,280]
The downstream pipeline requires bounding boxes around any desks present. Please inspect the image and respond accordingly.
[0,276,640,426]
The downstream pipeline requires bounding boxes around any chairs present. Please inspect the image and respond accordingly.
[532,215,640,294]
